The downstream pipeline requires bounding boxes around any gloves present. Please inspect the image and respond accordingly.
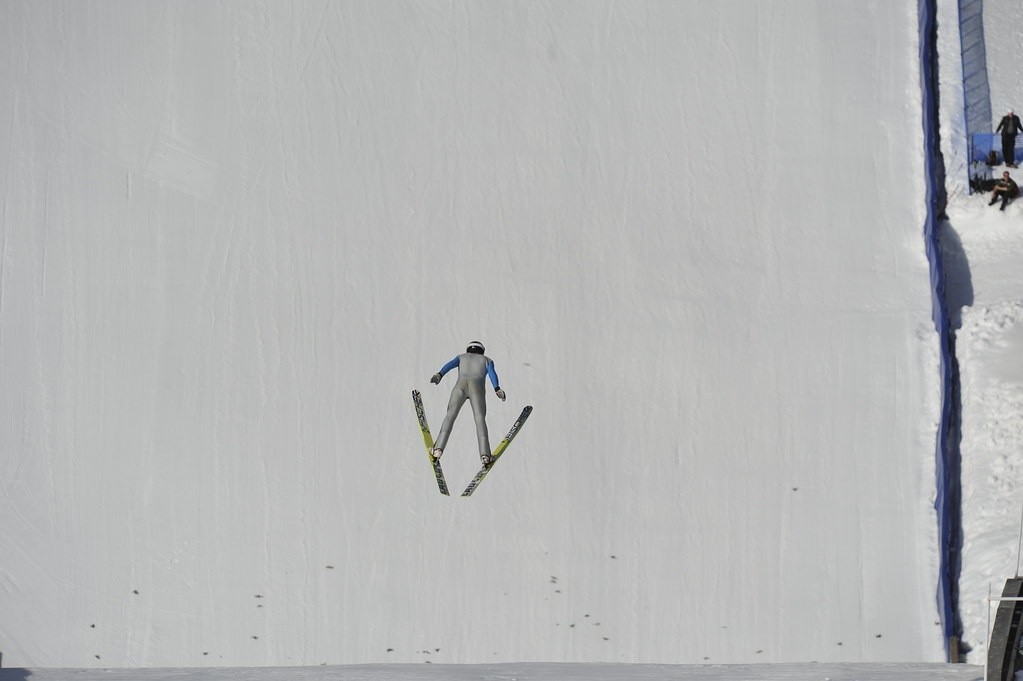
[495,387,506,401]
[430,372,443,385]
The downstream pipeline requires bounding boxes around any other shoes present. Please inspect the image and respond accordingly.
[433,449,442,458]
[481,453,495,465]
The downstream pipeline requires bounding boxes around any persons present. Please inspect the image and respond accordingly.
[995,108,1023,167]
[988,171,1019,211]
[428,341,506,466]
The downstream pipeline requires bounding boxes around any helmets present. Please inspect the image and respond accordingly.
[466,340,485,354]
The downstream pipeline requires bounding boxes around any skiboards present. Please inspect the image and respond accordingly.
[410,389,534,496]
